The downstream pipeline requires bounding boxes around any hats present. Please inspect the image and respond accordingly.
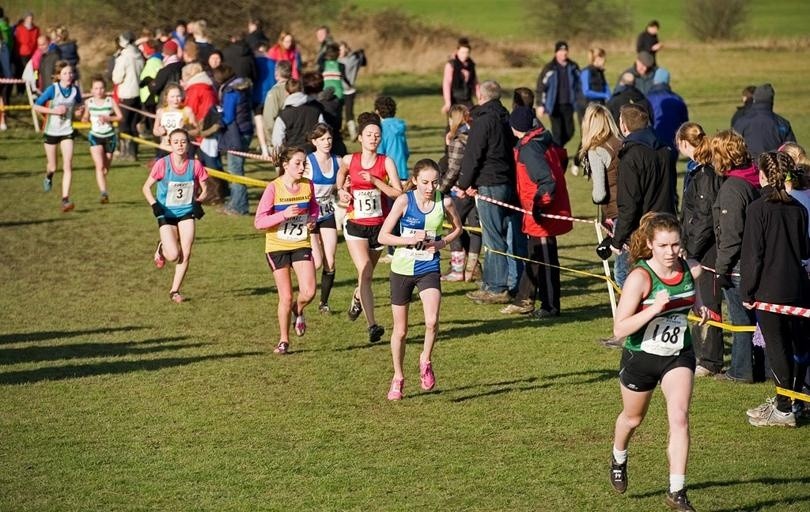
[116,31,201,57]
[506,104,535,133]
[741,80,776,102]
[632,49,674,86]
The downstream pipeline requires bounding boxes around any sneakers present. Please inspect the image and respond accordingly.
[662,486,697,512]
[743,394,799,430]
[385,375,406,401]
[417,351,436,391]
[367,323,383,343]
[608,449,629,496]
[38,151,141,211]
[153,238,188,303]
[439,248,564,319]
[273,339,289,356]
[347,286,365,320]
[289,299,332,338]
[690,364,757,385]
[196,189,250,219]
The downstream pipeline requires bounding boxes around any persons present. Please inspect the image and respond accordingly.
[254,95,461,401]
[611,212,710,512]
[436,17,809,428]
[1,10,364,303]
